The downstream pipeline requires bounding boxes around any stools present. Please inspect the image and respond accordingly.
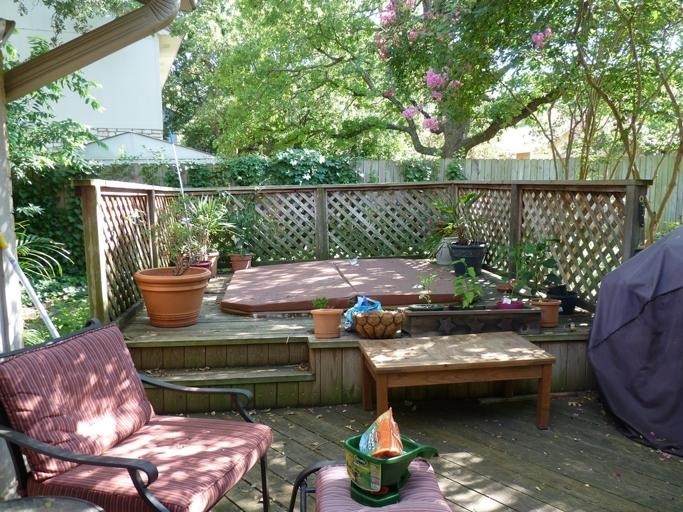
[286,455,452,512]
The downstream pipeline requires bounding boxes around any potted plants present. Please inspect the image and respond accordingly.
[125,194,253,329]
[424,190,488,276]
[406,233,579,330]
[311,297,343,339]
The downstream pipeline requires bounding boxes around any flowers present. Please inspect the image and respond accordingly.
[425,214,451,236]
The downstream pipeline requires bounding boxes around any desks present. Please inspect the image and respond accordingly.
[0,494,107,512]
[357,331,556,433]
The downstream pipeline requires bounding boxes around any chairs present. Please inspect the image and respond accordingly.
[0,320,273,512]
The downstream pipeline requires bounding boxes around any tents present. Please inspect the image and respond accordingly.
[586,223,681,458]
